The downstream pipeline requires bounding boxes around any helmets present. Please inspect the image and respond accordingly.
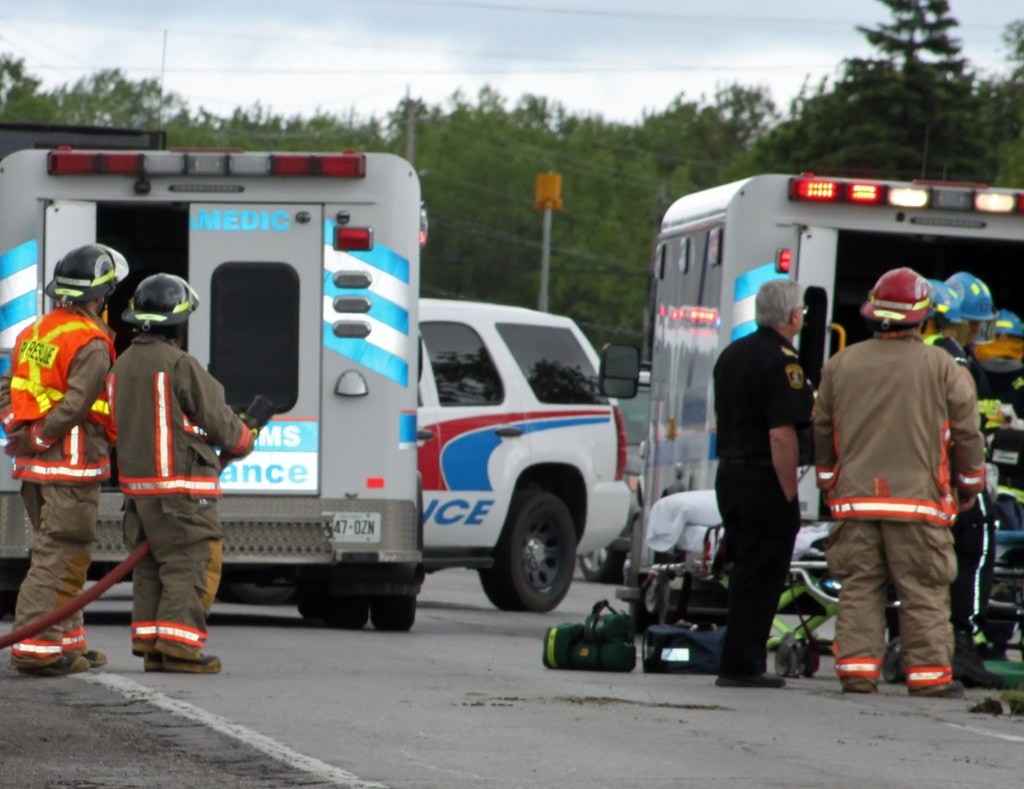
[121,272,200,325]
[860,266,934,324]
[44,243,130,302]
[923,271,996,323]
[975,308,1024,344]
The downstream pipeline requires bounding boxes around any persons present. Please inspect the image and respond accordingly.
[105,273,257,674]
[882,271,1024,689]
[0,244,129,676]
[814,266,985,697]
[713,279,815,688]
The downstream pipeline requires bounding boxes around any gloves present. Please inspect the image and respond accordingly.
[3,423,35,459]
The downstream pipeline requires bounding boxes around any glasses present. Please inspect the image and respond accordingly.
[799,304,809,315]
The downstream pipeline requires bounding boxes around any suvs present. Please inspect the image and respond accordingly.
[416,299,623,613]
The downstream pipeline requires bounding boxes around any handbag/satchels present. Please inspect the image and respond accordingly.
[641,623,728,675]
[540,599,637,673]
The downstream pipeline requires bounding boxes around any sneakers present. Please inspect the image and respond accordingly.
[839,678,879,694]
[18,649,108,676]
[143,651,222,674]
[909,679,964,699]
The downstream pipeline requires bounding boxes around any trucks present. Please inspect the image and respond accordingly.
[600,172,1023,658]
[1,146,433,632]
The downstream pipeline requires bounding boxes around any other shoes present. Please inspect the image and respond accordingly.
[714,672,786,689]
[953,655,1005,689]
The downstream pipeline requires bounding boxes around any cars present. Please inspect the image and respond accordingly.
[581,369,653,584]
